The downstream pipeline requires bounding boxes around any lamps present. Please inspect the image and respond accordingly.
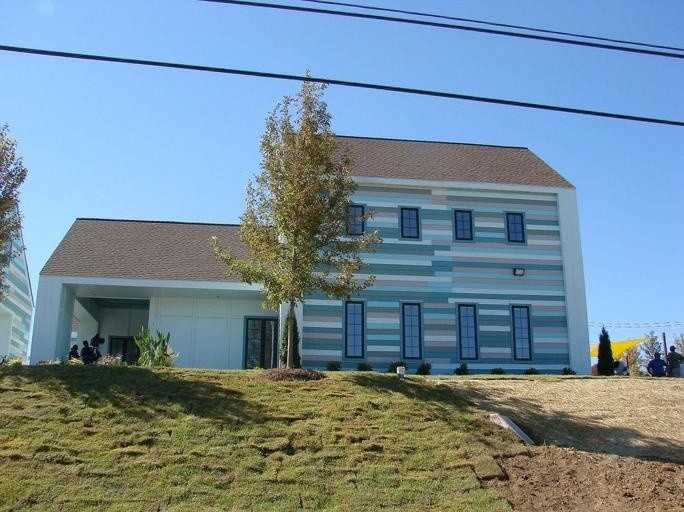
[514,266,526,278]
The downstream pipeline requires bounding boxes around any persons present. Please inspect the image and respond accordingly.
[95,350,101,361]
[665,346,684,373]
[647,352,668,377]
[81,340,97,364]
[614,360,630,376]
[69,344,80,360]
